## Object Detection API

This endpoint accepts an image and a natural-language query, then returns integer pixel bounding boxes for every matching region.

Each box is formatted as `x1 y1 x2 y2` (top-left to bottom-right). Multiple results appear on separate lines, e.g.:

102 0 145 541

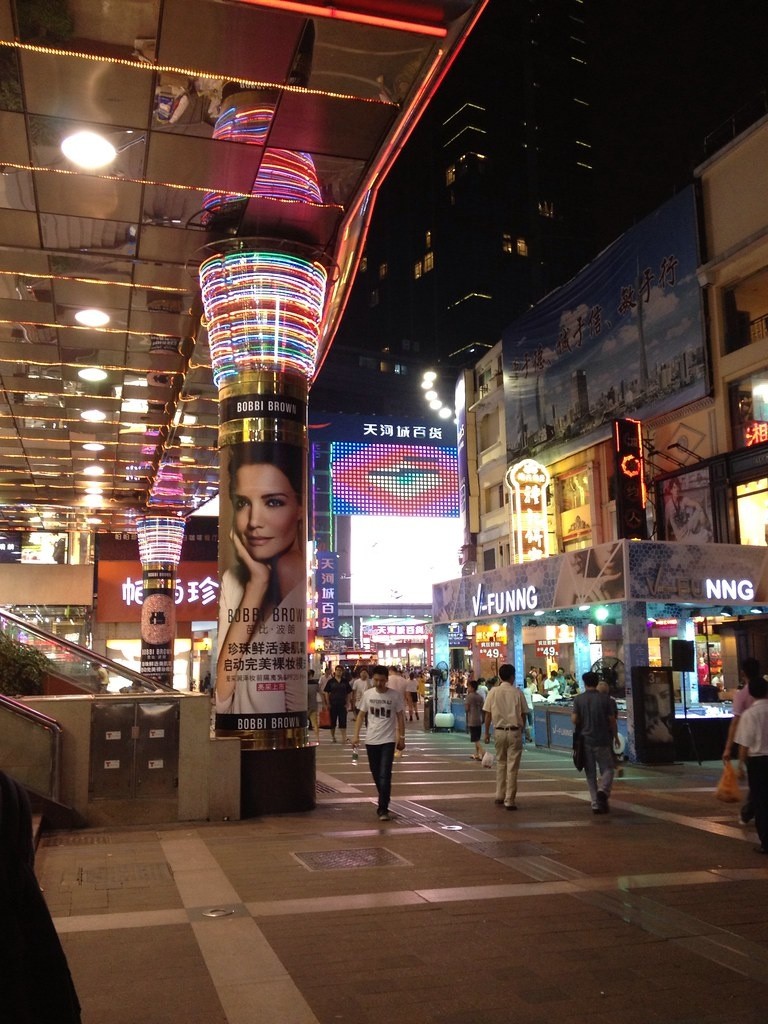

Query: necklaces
672 498 683 521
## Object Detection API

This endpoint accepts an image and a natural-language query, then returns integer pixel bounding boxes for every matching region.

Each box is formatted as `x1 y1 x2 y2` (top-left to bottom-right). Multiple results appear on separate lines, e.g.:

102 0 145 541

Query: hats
360 667 368 673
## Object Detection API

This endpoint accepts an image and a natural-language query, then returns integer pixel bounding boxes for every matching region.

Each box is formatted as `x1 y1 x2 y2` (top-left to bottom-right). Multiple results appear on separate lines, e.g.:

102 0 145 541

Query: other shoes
596 792 609 814
593 809 600 815
753 846 768 854
342 738 351 743
506 806 518 811
410 715 412 721
331 738 336 744
379 814 390 821
417 717 419 720
387 812 398 819
738 806 750 824
495 799 504 804
469 753 482 761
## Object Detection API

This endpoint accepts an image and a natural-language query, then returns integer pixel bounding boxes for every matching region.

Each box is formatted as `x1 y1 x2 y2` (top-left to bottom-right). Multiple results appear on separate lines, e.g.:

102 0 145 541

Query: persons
88 662 212 694
554 476 715 606
213 440 306 714
307 657 768 854
433 581 457 623
0 774 80 1024
645 673 673 755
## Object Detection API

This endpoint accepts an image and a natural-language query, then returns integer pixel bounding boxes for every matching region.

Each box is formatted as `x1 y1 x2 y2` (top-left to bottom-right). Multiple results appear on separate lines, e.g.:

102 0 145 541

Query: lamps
528 619 537 628
751 606 763 614
467 621 477 626
59 130 146 170
689 610 702 617
559 621 567 628
721 605 734 617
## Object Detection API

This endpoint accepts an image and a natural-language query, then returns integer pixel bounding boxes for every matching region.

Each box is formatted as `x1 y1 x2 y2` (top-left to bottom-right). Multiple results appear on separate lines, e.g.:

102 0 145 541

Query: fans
589 656 626 700
428 661 449 733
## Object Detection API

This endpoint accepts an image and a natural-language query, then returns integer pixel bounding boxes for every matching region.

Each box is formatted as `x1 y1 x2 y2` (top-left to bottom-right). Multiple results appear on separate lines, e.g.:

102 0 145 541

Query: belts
495 727 518 730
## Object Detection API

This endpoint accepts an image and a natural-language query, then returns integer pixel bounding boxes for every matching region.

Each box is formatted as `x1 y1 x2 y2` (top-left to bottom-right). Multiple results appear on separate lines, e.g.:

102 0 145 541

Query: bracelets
399 735 406 739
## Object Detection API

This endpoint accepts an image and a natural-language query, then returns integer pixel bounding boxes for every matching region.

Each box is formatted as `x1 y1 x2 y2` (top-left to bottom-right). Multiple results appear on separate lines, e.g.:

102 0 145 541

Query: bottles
352 744 358 766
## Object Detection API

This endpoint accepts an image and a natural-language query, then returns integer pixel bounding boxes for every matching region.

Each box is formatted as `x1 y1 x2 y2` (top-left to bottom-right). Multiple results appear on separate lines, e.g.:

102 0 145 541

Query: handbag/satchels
572 731 583 772
482 752 495 769
715 762 742 802
